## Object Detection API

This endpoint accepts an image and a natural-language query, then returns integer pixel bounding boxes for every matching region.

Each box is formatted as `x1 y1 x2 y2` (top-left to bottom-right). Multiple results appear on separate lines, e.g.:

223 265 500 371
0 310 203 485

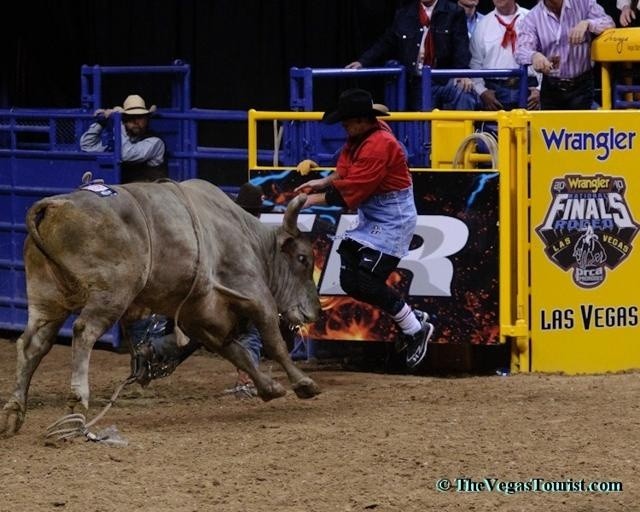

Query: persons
343 1 639 112
79 94 169 353
294 88 435 369
136 182 276 401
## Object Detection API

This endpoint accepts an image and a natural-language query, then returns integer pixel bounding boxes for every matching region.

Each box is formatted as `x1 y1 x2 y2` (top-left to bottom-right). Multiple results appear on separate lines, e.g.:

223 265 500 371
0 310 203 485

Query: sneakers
127 341 158 386
393 308 429 353
405 319 436 368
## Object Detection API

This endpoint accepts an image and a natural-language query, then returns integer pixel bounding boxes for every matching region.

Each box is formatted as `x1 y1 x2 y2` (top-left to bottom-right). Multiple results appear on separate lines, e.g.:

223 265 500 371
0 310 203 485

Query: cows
0 177 326 439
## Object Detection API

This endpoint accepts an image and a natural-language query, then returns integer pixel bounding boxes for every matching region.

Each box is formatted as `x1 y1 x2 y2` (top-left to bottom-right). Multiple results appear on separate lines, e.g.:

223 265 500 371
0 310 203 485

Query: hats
114 94 158 118
317 90 391 128
